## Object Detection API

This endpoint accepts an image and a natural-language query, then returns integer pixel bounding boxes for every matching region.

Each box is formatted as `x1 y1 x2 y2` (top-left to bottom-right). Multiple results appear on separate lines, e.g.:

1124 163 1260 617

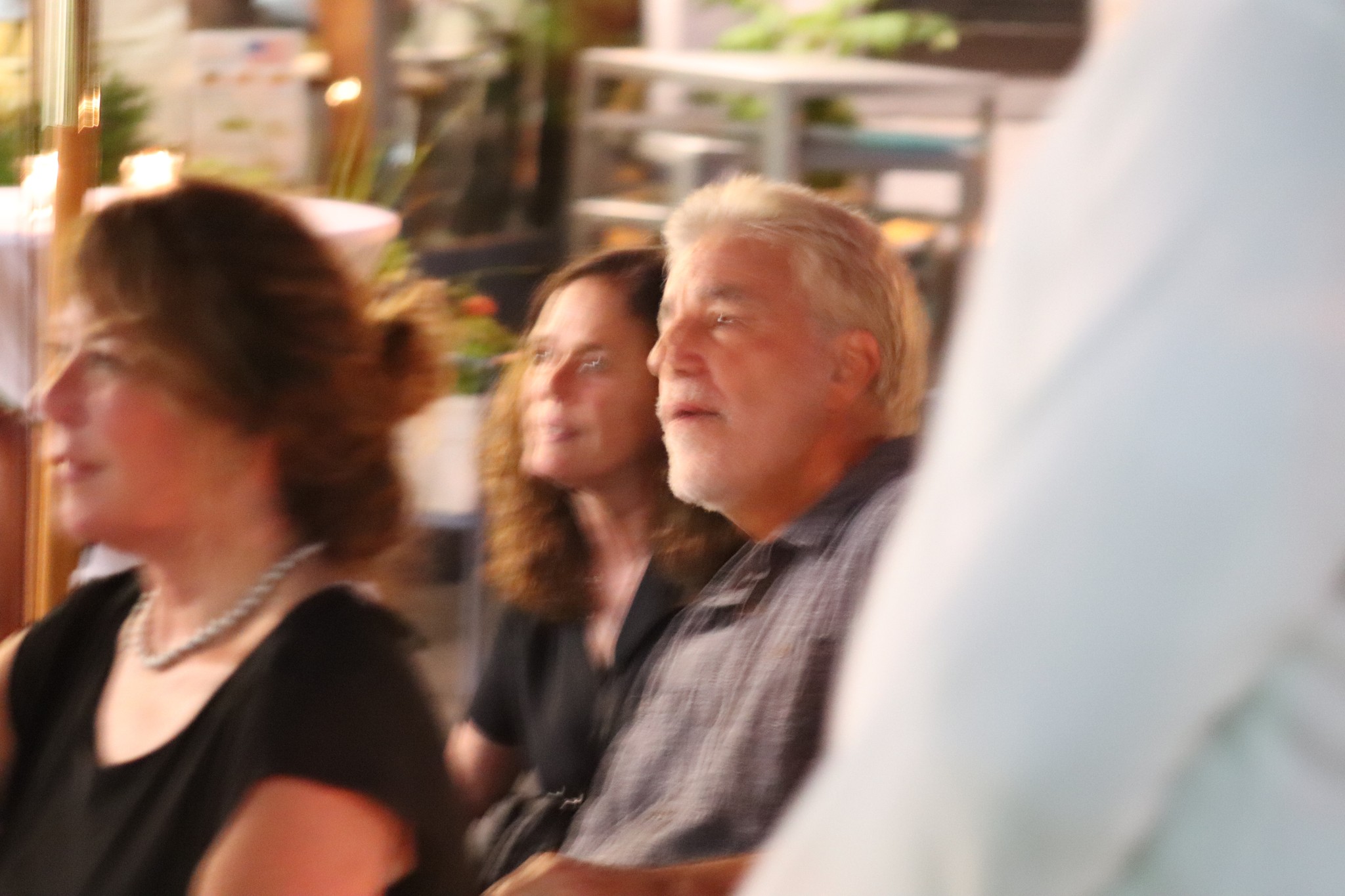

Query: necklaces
134 541 325 668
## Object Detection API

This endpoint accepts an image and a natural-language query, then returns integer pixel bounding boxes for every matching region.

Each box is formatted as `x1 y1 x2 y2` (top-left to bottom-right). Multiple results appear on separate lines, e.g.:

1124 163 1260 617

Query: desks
0 185 401 638
577 45 994 193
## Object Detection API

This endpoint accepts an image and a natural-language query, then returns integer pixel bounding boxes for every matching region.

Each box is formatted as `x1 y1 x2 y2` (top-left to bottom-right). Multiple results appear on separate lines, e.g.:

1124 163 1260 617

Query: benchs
569 198 955 257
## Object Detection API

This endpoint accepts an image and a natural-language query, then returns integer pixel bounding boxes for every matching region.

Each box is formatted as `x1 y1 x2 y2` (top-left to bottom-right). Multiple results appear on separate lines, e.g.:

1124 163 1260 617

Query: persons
726 0 1345 896
0 160 482 896
383 163 927 896
